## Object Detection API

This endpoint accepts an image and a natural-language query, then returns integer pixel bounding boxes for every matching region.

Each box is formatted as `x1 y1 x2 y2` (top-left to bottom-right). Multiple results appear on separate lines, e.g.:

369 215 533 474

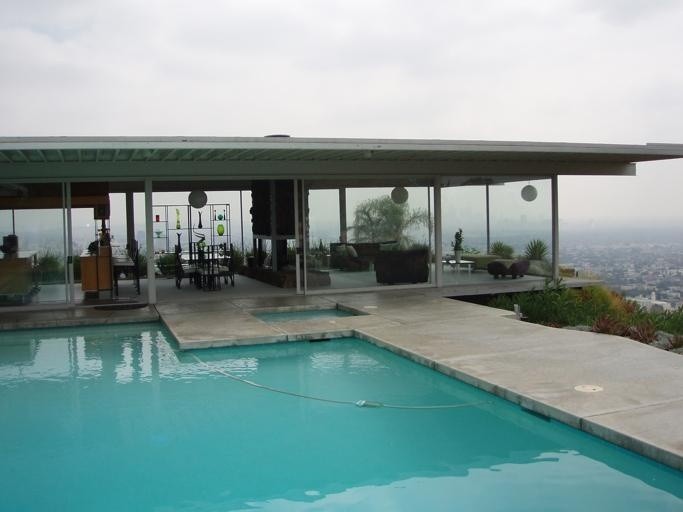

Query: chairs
112 238 142 297
173 240 235 293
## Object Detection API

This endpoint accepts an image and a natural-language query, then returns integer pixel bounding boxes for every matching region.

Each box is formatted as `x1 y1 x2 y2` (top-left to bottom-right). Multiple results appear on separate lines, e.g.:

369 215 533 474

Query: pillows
334 243 359 257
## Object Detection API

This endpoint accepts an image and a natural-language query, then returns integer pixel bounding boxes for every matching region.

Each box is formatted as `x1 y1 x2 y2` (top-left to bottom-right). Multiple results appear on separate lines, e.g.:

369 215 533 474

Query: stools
487 261 528 280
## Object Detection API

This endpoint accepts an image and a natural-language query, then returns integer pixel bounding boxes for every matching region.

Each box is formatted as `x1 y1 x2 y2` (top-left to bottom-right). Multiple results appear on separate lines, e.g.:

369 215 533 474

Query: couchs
374 247 430 285
329 242 400 272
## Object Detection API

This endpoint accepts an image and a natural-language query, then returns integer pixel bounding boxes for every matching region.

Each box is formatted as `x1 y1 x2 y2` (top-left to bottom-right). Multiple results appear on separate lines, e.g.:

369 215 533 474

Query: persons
175 245 185 288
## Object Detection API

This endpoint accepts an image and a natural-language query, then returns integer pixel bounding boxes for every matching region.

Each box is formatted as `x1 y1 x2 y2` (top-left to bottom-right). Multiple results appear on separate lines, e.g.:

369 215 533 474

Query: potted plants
448 226 467 262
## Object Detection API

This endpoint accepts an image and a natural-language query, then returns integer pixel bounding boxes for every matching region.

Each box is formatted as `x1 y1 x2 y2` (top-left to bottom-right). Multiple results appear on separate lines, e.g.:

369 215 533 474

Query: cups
197 243 203 251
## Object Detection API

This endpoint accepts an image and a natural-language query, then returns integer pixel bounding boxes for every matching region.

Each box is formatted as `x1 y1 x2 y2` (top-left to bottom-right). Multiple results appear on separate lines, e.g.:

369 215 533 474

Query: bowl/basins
155 231 162 237
112 254 129 263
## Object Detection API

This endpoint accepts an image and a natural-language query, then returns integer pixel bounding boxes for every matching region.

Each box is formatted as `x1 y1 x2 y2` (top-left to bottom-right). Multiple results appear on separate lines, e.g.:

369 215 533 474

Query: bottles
216 224 224 235
155 215 158 221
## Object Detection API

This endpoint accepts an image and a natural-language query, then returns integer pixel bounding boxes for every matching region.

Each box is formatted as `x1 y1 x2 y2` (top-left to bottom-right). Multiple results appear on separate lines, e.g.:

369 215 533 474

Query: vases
217 225 225 236
175 209 180 229
198 210 203 228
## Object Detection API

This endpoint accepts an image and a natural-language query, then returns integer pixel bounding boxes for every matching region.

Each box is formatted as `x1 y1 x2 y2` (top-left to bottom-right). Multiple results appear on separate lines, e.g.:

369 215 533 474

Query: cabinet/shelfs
150 203 233 253
0 249 44 308
